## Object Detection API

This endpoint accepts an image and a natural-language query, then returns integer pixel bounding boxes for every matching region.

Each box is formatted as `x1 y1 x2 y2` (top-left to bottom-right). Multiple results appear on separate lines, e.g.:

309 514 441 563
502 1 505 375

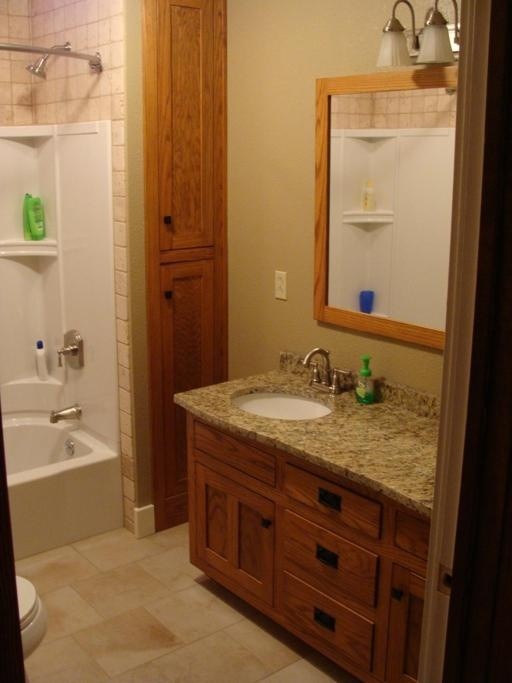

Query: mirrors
313 66 463 351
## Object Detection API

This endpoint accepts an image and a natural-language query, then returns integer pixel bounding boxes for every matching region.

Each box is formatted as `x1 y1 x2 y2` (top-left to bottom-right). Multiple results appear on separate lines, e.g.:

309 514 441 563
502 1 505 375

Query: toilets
16 573 48 662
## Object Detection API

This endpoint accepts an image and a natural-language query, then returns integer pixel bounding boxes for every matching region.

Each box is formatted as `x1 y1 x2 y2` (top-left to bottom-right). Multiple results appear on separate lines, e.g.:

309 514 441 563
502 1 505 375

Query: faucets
49 403 81 424
302 347 332 389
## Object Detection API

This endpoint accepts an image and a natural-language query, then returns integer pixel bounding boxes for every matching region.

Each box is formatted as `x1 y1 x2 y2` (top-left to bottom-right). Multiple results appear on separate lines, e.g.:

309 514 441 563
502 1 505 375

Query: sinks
229 384 333 419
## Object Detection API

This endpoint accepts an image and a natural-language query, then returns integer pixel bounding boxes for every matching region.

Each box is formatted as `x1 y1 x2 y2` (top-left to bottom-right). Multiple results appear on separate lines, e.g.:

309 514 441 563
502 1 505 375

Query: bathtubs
2 413 123 563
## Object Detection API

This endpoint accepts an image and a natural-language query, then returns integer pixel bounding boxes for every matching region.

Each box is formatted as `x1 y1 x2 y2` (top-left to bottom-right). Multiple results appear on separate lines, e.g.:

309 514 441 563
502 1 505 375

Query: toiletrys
362 177 377 212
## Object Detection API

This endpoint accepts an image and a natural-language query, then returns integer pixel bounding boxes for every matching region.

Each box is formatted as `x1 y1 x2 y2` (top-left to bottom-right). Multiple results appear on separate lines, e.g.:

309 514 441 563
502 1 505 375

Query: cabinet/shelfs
186 409 431 682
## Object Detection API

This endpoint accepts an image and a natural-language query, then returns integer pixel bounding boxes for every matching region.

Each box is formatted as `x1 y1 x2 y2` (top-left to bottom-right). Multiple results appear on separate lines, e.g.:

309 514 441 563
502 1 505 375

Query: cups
359 290 373 313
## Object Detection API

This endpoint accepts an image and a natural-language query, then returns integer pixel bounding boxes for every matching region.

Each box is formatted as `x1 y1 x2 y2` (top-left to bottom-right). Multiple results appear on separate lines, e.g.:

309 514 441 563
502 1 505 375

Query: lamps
376 0 461 68
